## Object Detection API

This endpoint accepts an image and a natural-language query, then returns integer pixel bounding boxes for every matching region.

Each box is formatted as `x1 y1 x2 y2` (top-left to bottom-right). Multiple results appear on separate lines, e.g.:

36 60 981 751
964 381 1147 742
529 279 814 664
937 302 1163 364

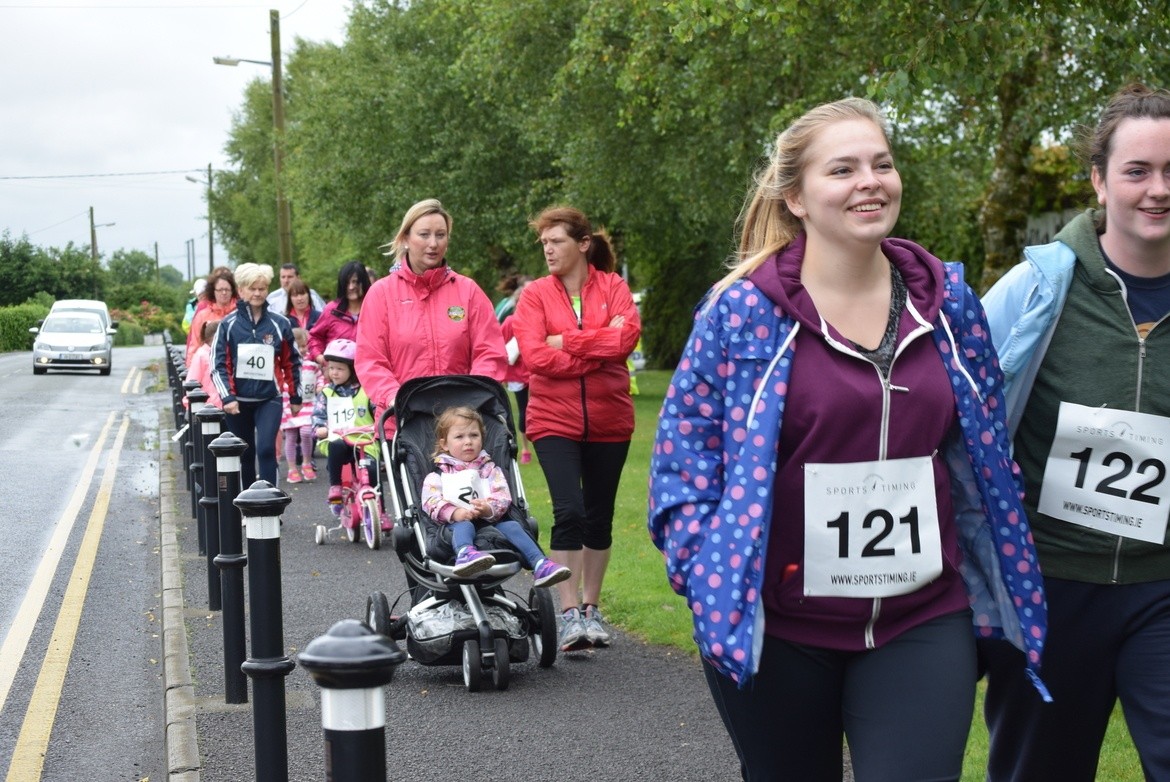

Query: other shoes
379 514 393 530
328 484 342 499
310 458 318 471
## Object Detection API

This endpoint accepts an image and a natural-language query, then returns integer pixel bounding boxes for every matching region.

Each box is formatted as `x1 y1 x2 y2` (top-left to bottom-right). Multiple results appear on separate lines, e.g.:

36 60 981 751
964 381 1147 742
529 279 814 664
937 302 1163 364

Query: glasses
215 287 232 293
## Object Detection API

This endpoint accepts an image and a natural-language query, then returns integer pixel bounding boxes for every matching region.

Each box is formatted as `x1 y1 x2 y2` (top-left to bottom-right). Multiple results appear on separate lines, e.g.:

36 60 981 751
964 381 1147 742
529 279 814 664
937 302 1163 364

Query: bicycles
314 428 390 551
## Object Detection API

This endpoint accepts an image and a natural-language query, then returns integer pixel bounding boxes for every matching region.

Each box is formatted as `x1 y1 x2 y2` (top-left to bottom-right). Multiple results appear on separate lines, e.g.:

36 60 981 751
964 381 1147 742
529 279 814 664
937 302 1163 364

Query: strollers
377 374 558 693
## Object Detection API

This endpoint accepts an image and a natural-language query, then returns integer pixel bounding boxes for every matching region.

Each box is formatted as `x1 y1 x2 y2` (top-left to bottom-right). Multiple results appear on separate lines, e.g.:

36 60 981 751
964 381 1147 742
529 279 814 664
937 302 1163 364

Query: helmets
323 339 357 366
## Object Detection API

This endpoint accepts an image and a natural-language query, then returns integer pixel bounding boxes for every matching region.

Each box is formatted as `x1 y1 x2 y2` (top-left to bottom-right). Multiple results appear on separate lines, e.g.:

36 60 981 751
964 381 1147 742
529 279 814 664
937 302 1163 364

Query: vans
38 298 119 352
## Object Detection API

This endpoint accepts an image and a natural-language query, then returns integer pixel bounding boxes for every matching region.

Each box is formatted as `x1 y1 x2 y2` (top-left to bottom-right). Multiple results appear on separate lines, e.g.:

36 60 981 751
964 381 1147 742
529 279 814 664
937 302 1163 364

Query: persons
208 263 305 503
182 258 379 485
308 337 397 533
496 205 642 652
646 94 1049 781
423 408 575 592
352 195 505 623
962 73 1170 782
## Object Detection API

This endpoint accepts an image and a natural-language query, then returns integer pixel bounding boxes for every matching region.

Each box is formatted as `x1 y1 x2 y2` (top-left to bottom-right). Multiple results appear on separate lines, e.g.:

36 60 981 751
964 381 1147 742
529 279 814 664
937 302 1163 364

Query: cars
28 312 117 376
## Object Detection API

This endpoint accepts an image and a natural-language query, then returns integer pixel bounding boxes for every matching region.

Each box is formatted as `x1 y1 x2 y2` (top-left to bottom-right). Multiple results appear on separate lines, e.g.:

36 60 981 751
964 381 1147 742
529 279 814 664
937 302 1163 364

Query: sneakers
286 468 303 484
302 463 317 480
581 603 612 647
557 605 592 651
532 559 571 587
453 546 497 577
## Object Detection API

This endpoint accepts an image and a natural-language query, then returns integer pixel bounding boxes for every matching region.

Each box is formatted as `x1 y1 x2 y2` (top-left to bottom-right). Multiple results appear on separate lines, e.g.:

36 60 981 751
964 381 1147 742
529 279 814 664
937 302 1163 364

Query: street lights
212 54 294 266
185 175 213 273
91 223 117 300
